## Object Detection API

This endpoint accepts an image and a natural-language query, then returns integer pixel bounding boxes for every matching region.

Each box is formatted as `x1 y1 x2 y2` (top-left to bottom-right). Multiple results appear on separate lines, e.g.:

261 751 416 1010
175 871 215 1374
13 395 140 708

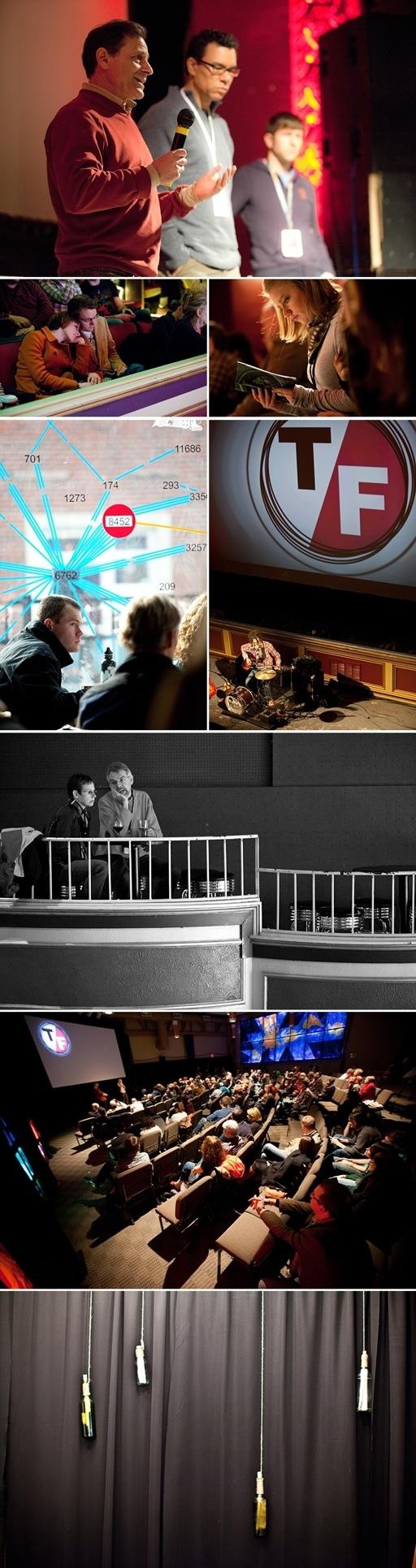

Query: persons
0 595 89 730
94 761 179 900
231 112 337 277
77 592 206 730
87 1067 416 1289
47 773 125 900
241 630 282 694
44 19 237 277
0 279 416 416
137 27 241 277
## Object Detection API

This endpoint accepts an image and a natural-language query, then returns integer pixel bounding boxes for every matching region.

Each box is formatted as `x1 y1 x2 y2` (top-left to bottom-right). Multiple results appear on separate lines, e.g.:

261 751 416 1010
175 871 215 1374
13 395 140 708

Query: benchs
1 322 153 405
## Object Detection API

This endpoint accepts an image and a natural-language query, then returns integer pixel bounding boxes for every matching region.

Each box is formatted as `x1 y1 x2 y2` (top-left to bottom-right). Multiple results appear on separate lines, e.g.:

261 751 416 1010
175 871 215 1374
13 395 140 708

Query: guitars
241 654 290 671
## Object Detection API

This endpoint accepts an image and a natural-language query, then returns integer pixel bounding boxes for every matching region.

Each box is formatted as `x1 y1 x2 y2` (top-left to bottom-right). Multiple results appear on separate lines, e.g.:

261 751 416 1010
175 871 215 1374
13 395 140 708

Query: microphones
160 108 195 188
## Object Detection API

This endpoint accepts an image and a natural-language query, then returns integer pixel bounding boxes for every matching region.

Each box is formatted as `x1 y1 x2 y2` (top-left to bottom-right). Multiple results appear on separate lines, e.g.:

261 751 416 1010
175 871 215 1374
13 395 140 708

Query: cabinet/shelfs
124 280 163 318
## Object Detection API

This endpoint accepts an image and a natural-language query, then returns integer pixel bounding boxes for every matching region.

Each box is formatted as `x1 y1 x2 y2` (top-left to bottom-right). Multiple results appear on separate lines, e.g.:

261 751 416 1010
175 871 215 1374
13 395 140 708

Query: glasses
79 790 98 794
199 59 240 78
79 313 99 322
309 1191 322 1205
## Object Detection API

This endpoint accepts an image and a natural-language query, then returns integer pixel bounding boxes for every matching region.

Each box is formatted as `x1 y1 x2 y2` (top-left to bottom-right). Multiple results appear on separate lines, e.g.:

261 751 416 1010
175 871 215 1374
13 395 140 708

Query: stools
133 873 151 898
60 868 235 897
289 899 398 932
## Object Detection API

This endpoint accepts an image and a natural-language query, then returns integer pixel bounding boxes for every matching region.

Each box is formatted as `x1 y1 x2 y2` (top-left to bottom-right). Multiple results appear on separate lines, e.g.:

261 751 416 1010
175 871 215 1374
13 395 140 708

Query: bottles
82 1374 96 1441
253 1471 267 1535
134 1345 150 1386
358 1351 373 1412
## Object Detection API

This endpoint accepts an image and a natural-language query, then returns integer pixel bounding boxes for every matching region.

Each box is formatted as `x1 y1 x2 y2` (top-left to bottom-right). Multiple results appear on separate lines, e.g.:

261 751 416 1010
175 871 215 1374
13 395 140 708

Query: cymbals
255 669 276 680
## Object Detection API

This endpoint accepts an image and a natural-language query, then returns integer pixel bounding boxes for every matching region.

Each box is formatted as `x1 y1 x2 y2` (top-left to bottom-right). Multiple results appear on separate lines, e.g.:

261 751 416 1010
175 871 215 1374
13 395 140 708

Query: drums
224 685 254 715
